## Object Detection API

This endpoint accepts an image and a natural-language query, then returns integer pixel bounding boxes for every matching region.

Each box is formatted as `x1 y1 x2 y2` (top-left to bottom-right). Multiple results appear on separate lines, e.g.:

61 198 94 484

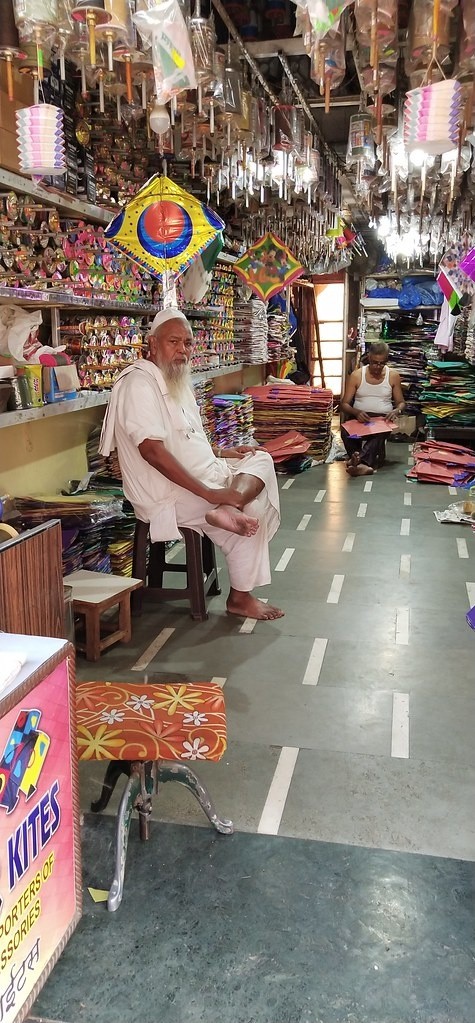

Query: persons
97 308 285 620
340 343 406 476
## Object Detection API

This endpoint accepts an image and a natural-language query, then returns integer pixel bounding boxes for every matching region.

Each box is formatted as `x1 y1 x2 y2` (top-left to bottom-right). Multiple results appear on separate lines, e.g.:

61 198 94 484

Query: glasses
369 358 385 367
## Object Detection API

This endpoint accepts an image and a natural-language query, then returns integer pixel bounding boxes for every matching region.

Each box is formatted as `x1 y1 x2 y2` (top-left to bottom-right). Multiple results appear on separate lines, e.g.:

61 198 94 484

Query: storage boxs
37 360 80 404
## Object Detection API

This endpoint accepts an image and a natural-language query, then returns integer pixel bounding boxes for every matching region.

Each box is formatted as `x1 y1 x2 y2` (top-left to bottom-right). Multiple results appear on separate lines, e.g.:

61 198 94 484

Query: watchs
397 408 403 413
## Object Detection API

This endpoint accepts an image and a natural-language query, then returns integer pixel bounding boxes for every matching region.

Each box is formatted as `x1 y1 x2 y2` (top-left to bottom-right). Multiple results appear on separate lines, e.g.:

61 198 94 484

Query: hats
147 309 195 336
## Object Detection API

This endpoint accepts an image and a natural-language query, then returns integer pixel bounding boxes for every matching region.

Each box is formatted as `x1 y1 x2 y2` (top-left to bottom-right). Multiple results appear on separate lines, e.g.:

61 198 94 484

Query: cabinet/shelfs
1 0 245 430
356 270 450 372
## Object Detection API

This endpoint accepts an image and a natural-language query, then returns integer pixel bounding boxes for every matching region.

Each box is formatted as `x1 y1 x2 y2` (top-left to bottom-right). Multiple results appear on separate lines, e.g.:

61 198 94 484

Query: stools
64 568 145 665
67 680 233 913
130 518 221 625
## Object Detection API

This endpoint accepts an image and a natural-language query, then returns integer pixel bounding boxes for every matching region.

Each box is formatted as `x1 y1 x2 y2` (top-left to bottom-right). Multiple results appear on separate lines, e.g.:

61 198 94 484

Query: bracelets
218 449 223 457
